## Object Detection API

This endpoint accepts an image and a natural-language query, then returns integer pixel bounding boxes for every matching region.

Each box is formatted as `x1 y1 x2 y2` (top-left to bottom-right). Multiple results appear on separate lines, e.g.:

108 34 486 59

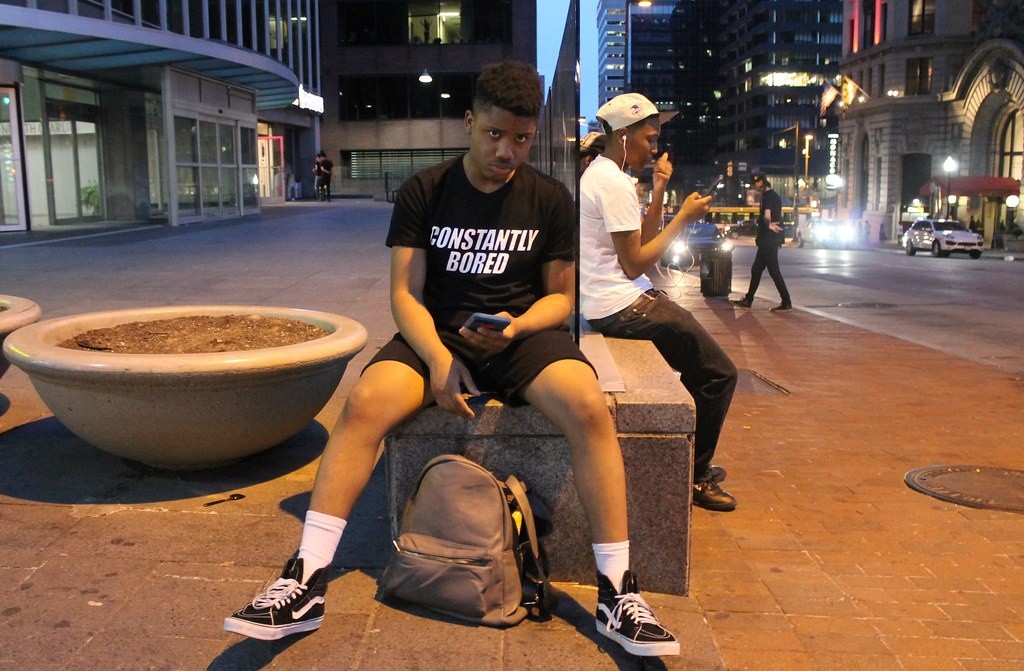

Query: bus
705 207 821 240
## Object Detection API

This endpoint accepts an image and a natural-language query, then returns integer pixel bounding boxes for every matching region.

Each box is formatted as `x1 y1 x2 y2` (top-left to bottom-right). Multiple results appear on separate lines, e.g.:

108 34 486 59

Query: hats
595 92 680 133
579 129 609 158
748 170 766 184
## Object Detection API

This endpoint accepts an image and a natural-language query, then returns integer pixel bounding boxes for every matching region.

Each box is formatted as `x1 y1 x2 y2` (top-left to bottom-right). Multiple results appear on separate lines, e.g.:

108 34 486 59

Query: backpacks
379 453 553 629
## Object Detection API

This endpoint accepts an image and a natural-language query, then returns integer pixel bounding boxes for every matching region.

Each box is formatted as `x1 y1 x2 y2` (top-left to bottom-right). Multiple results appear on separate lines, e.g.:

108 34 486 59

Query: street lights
805 134 815 180
623 0 653 93
356 104 372 121
944 155 959 219
420 66 444 164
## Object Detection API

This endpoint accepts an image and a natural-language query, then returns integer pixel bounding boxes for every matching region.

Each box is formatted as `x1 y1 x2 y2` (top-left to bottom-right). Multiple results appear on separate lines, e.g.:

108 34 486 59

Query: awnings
918 177 1020 217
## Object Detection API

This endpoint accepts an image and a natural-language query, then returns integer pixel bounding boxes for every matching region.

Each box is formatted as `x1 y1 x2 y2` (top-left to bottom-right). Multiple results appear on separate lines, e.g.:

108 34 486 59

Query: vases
0 293 367 469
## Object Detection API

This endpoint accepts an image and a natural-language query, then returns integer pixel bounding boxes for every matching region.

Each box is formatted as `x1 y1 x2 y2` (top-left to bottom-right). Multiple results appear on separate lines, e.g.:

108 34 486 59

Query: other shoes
709 465 726 481
693 477 739 509
768 304 792 312
729 296 751 310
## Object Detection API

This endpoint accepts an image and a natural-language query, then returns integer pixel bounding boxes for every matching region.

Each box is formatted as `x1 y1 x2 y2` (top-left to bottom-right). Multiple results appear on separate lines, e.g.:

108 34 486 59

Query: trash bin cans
699 244 733 297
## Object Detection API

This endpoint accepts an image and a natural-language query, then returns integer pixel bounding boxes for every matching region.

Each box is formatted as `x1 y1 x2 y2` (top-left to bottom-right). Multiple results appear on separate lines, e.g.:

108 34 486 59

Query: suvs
684 223 732 265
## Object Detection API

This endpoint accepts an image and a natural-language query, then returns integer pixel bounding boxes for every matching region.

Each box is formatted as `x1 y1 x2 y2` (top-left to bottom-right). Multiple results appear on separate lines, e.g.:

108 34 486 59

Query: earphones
620 136 627 146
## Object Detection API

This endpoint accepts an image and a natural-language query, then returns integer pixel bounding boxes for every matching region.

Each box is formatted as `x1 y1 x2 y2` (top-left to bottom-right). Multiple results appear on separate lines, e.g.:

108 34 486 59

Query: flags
840 75 858 118
819 81 839 116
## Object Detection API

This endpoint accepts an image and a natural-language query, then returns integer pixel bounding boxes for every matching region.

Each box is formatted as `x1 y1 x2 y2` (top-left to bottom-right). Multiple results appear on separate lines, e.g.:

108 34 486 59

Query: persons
896 222 903 246
863 220 871 244
221 63 681 657
968 214 976 232
580 91 738 512
311 150 334 202
727 172 793 312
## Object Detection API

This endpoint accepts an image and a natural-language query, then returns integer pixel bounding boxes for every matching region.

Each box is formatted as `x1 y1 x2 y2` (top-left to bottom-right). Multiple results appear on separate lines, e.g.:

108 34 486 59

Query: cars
726 219 759 239
903 218 984 259
797 218 854 249
664 213 675 227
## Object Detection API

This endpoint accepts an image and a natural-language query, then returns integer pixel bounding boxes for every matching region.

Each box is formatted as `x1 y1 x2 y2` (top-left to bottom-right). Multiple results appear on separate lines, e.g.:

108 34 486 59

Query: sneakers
594 568 680 656
222 549 332 641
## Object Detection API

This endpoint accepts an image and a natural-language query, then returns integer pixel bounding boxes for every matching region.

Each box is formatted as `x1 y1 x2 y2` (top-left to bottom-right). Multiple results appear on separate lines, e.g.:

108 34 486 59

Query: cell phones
701 175 724 197
464 313 511 334
